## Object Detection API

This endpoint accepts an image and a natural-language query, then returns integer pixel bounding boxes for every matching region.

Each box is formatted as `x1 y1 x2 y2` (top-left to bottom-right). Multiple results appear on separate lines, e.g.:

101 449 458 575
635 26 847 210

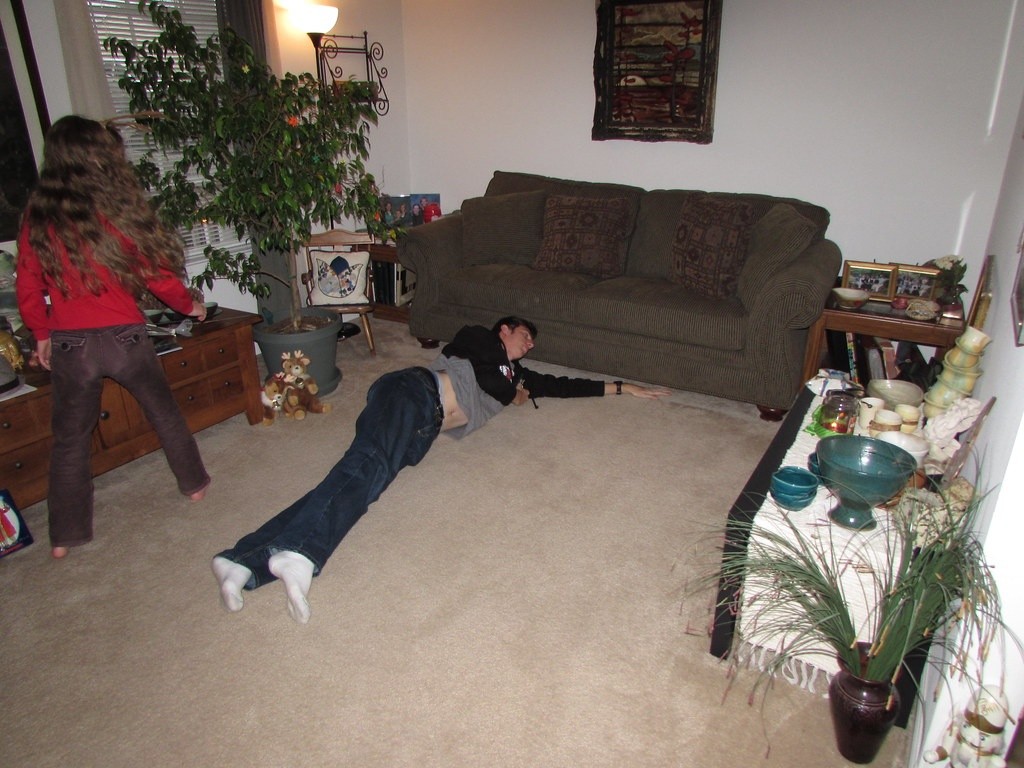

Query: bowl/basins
140 310 163 324
767 465 820 512
867 379 924 412
831 287 872 311
164 302 218 322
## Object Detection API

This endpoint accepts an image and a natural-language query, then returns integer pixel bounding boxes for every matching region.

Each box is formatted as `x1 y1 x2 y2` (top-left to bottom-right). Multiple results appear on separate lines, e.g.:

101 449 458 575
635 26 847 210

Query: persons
19 117 213 557
385 198 439 231
212 315 671 623
857 273 923 296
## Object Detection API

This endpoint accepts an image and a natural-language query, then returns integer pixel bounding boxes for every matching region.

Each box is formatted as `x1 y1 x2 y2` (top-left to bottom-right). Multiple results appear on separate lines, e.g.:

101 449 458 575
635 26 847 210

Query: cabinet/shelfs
0 307 264 508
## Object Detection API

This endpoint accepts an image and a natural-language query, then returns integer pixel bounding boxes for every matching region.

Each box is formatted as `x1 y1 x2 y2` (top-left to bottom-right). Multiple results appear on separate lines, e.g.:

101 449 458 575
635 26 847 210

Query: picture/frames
841 260 941 303
1009 242 1024 346
967 255 993 331
591 0 723 145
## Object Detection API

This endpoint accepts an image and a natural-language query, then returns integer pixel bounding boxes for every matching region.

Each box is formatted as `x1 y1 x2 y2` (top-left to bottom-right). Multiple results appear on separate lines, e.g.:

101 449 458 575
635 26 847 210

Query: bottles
817 388 861 437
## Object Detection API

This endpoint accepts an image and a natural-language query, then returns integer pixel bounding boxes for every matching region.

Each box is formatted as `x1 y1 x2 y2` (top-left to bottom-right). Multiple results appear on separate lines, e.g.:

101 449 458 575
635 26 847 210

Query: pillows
666 193 754 300
461 190 546 268
533 194 641 279
736 201 817 312
306 251 371 306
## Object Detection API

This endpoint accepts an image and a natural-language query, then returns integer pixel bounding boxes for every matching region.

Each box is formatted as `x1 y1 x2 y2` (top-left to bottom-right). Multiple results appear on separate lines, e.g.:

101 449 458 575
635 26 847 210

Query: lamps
290 6 361 340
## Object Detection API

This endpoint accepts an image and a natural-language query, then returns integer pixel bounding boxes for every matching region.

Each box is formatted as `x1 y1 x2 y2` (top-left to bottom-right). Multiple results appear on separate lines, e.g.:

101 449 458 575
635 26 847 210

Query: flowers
933 255 968 299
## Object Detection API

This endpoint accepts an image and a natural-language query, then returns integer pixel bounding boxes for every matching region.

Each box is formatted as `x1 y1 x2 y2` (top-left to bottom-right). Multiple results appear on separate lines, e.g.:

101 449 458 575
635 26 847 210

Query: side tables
356 229 411 325
800 277 966 397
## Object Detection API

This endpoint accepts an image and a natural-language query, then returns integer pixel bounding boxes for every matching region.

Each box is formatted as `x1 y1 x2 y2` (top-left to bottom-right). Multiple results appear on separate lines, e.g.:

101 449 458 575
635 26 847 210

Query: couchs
397 169 842 422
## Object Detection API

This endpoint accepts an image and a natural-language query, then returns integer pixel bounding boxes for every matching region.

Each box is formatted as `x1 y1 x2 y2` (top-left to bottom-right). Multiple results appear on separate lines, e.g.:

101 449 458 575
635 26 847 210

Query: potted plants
103 0 382 400
665 436 1024 763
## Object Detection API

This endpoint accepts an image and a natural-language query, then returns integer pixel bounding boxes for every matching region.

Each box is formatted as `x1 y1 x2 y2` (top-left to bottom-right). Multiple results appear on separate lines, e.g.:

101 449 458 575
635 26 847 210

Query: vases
941 285 967 319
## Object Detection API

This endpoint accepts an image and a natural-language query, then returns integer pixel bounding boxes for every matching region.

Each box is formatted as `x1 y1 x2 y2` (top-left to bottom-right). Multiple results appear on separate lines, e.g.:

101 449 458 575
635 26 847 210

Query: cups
922 325 993 423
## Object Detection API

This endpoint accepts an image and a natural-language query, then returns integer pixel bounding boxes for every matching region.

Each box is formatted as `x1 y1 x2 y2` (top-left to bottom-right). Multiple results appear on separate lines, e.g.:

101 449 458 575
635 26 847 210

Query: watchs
613 380 624 395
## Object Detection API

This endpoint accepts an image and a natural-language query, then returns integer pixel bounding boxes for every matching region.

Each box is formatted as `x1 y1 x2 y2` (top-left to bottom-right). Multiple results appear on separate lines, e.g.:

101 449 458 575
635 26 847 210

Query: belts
415 364 444 434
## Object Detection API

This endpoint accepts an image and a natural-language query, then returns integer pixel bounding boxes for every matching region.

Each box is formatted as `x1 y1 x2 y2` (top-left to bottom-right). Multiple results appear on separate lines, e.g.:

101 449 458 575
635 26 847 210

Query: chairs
302 229 378 357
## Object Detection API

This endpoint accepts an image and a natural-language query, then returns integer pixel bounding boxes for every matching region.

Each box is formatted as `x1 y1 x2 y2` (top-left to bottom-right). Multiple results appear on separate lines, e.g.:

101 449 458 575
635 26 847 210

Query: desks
709 386 956 728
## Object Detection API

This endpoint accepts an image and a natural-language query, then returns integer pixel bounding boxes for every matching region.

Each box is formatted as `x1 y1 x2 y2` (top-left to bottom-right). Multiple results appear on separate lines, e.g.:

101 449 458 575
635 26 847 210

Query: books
845 332 900 387
